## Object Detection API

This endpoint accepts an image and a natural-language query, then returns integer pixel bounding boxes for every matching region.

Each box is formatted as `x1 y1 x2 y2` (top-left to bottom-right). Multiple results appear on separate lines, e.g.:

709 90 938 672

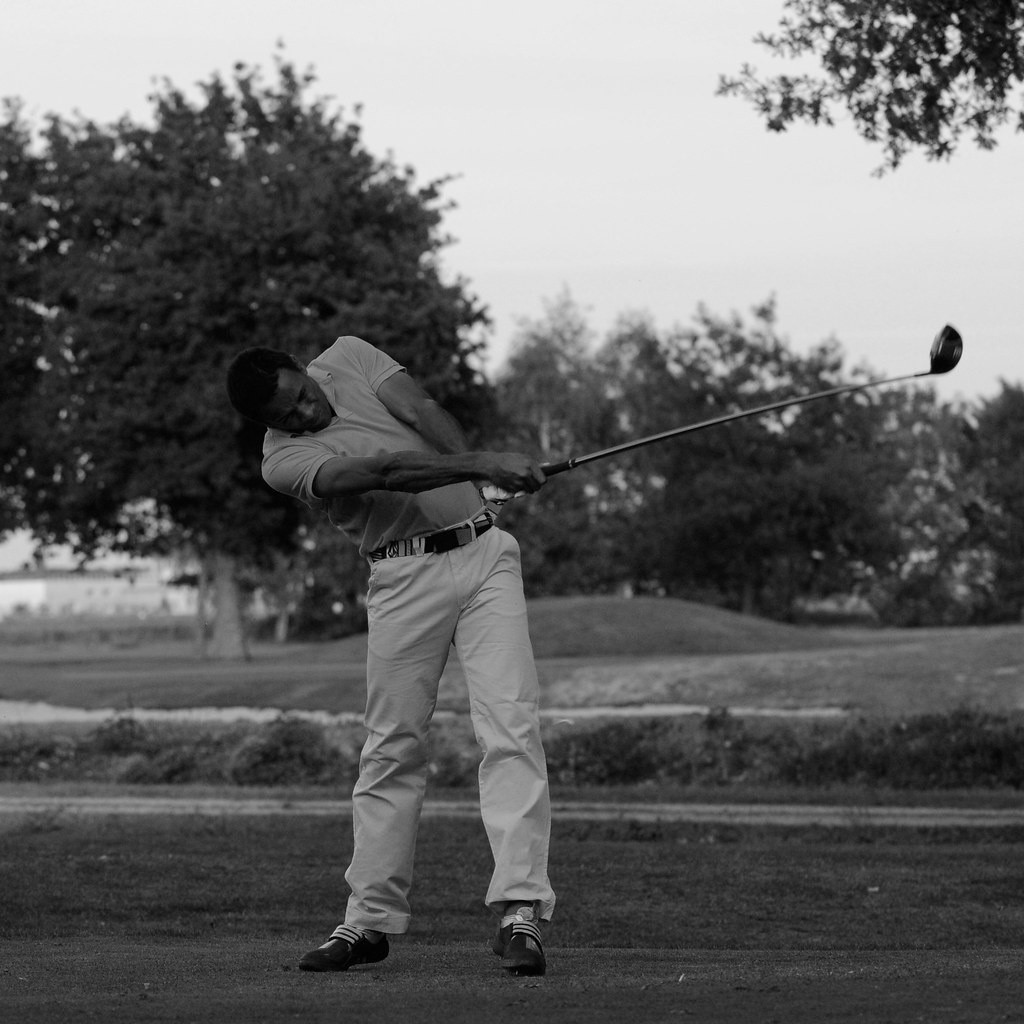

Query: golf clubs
478 323 964 501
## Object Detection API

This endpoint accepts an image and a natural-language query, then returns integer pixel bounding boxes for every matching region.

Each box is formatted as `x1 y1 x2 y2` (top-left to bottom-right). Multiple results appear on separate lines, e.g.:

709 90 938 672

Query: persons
229 334 557 975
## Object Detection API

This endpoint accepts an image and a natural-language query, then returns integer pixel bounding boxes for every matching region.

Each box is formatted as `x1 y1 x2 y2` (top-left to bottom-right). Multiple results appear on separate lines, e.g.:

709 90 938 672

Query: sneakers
298 924 389 972
492 907 547 977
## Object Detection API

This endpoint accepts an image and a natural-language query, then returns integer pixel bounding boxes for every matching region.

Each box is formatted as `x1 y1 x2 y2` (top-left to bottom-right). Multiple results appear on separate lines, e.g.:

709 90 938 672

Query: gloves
481 485 527 504
